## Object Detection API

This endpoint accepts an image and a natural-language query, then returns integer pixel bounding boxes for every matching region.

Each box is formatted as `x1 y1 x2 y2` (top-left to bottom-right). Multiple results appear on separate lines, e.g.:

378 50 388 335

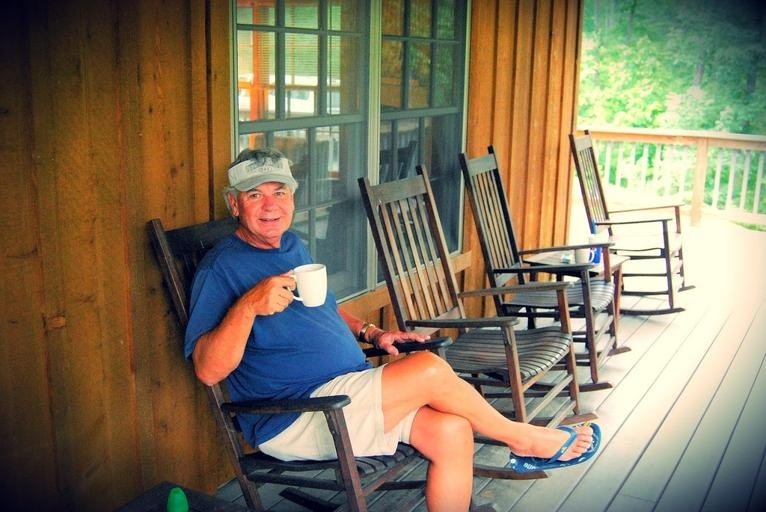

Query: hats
227 157 299 194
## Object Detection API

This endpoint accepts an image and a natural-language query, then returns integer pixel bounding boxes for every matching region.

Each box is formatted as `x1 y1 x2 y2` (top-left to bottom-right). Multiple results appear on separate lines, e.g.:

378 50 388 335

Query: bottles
589 219 602 264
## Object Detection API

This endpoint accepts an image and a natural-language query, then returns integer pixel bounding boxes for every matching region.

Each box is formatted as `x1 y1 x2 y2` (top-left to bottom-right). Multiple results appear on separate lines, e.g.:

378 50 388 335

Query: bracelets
358 319 377 345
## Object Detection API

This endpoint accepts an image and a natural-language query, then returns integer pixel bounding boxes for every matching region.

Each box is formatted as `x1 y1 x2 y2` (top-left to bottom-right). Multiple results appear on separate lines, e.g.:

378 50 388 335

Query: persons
183 146 602 512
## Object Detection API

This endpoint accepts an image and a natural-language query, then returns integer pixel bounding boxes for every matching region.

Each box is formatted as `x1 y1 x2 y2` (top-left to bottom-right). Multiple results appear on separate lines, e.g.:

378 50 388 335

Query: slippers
509 421 601 474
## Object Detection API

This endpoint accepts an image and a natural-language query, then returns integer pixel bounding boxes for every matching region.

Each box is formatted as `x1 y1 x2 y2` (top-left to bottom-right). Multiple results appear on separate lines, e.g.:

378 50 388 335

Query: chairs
357 163 597 448
147 216 548 511
459 144 631 392
569 130 695 316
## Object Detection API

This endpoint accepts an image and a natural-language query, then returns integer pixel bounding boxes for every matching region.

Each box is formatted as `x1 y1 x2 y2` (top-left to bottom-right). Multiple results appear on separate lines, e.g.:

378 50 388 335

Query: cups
286 263 328 308
573 248 595 263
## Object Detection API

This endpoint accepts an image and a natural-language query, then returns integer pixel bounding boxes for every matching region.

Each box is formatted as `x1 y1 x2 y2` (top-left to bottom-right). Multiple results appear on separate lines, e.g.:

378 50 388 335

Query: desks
113 481 262 512
